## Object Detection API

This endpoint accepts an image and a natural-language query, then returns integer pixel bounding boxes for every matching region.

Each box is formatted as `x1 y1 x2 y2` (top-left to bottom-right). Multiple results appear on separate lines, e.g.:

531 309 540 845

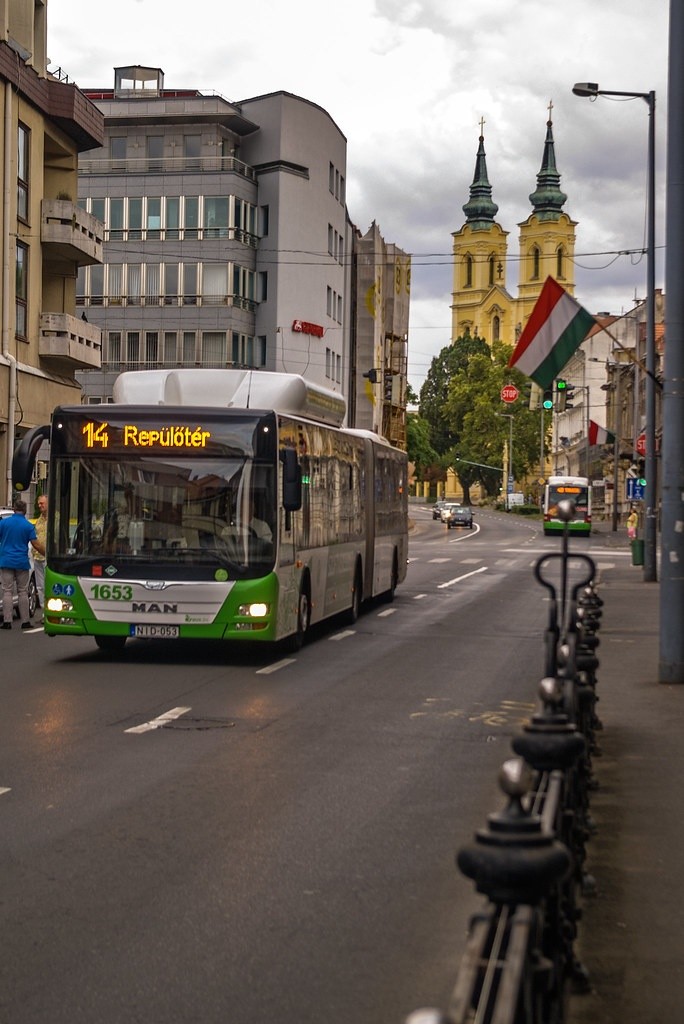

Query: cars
446 505 476 529
0 506 39 619
431 501 450 520
439 502 461 523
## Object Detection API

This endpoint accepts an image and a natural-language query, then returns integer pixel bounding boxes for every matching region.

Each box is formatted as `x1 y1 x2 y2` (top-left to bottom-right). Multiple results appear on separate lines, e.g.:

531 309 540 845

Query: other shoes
21 621 34 629
0 622 12 629
41 617 44 624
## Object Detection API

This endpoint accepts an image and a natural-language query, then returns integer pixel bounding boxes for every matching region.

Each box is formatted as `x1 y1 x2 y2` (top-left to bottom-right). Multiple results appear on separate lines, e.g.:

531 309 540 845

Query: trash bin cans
631 540 644 565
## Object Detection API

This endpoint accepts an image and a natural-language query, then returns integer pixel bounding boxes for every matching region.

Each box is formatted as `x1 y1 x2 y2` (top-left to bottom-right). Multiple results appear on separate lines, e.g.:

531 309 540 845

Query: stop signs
635 433 659 458
501 385 518 403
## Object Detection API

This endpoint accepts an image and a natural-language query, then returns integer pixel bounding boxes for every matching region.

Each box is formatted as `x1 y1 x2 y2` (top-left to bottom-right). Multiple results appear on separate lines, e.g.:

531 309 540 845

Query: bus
11 367 410 654
541 476 594 536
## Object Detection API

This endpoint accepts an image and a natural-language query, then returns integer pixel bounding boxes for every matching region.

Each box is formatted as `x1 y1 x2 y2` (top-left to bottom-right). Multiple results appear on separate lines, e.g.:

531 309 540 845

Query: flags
589 419 616 446
508 275 597 391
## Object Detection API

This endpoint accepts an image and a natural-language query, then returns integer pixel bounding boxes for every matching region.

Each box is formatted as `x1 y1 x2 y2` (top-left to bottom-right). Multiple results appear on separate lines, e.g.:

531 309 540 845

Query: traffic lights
362 368 377 383
456 451 460 462
556 378 566 392
637 458 647 487
542 390 553 410
522 383 539 411
559 384 575 412
384 375 401 407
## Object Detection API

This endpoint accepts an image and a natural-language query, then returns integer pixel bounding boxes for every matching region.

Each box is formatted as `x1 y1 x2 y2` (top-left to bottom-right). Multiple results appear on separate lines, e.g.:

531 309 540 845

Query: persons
221 496 272 542
0 500 46 630
31 495 60 622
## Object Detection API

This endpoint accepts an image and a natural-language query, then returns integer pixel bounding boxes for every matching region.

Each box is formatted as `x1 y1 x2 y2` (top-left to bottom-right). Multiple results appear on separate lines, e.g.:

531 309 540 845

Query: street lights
571 81 659 586
501 413 513 494
